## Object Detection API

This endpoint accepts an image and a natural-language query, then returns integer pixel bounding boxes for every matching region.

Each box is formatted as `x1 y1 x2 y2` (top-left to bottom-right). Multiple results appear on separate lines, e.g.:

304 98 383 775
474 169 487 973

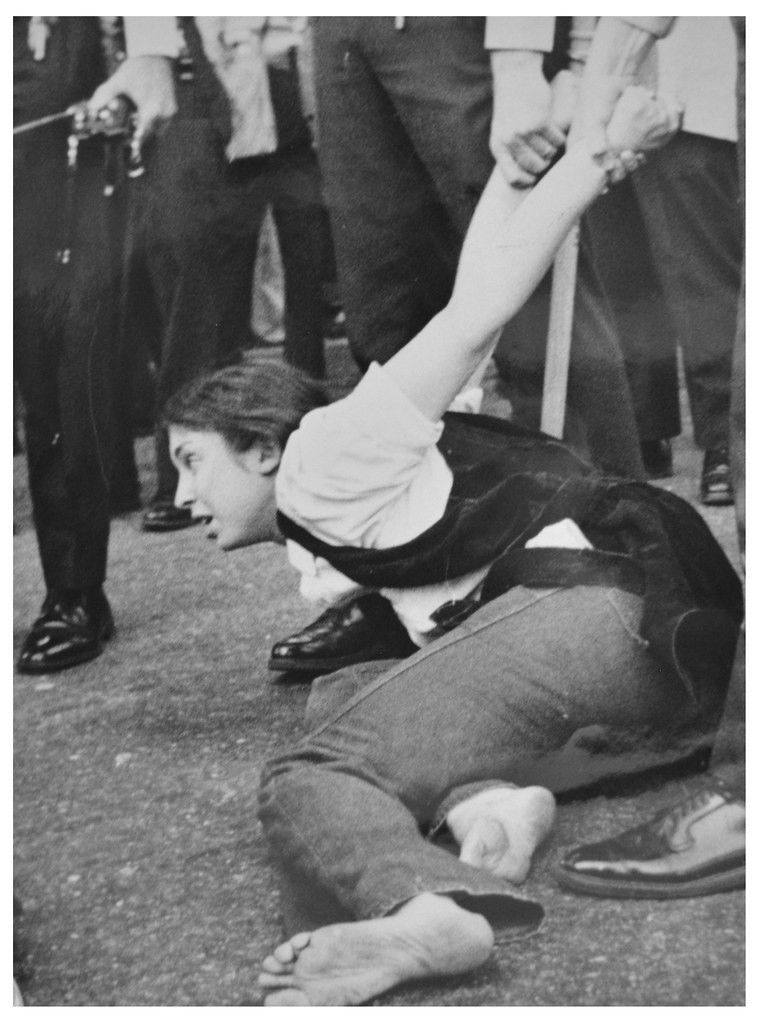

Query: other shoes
322 311 348 341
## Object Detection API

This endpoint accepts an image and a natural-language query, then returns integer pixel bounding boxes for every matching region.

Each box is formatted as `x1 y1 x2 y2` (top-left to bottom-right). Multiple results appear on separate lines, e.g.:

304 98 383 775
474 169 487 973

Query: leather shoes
550 772 746 899
141 493 195 529
702 442 734 504
16 584 113 670
638 435 672 478
269 593 424 672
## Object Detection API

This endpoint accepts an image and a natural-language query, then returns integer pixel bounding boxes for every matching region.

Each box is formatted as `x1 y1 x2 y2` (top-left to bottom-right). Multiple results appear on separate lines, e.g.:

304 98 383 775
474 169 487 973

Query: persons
102 16 734 674
481 18 746 895
162 69 746 1005
13 18 189 672
